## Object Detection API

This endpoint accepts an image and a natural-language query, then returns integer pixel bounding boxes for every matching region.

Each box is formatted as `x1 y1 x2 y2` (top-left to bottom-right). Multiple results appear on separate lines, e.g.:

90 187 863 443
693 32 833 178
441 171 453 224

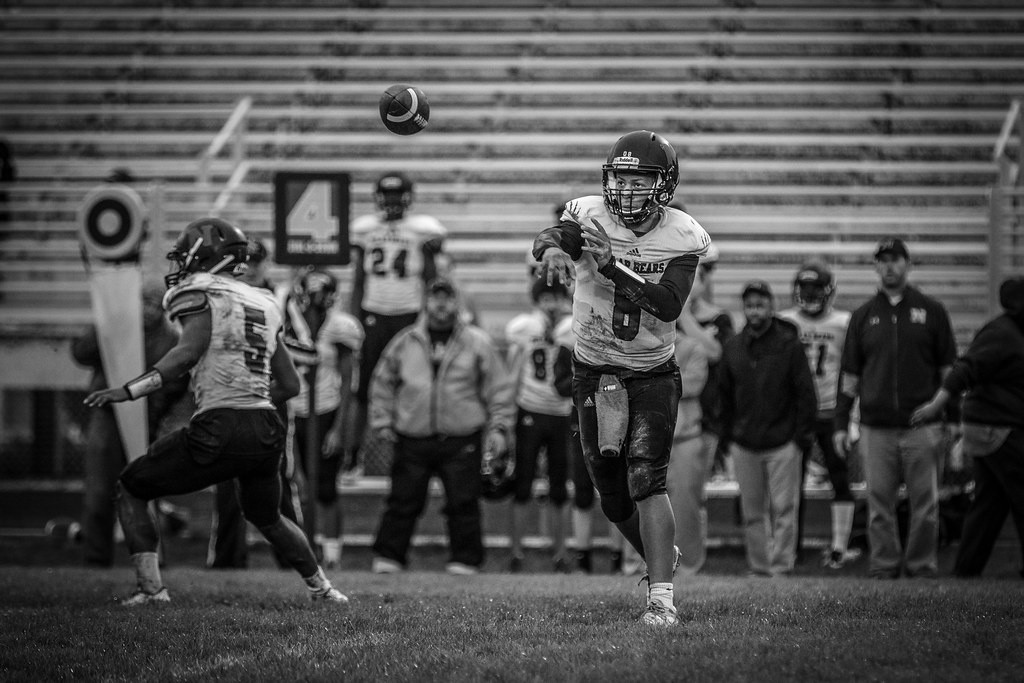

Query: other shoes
509 558 522 572
830 548 862 569
554 559 570 573
323 560 341 571
372 559 400 572
444 561 479 576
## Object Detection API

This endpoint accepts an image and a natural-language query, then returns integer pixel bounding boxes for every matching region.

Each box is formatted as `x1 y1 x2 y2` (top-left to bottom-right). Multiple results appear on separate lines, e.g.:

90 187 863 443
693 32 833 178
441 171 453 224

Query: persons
911 277 1023 578
683 252 734 475
345 171 436 471
290 270 366 568
83 218 348 608
775 267 864 576
507 275 576 571
529 129 710 624
366 283 517 574
618 335 710 574
833 239 959 578
204 238 330 571
716 283 817 576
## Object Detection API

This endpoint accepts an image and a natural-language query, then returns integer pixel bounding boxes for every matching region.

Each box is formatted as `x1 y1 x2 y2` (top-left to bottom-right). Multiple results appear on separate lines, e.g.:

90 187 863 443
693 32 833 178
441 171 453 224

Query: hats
740 279 776 302
873 238 910 260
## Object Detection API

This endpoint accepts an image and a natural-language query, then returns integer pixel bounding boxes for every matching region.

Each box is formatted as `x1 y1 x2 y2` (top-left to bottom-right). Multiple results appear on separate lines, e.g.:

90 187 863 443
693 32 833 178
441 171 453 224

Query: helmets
791 265 835 317
294 270 338 309
373 173 412 223
529 268 568 303
600 130 678 226
421 277 462 327
246 238 267 272
163 219 251 289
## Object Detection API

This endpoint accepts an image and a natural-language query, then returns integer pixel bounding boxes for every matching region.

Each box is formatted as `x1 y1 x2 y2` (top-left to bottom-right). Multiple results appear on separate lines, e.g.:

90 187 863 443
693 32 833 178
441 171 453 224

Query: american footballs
379 85 430 135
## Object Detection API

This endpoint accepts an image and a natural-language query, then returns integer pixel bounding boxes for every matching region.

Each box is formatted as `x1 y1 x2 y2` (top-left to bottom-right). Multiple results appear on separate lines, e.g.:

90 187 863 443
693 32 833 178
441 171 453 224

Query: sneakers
119 586 170 605
307 580 349 605
636 599 680 626
637 545 683 605
